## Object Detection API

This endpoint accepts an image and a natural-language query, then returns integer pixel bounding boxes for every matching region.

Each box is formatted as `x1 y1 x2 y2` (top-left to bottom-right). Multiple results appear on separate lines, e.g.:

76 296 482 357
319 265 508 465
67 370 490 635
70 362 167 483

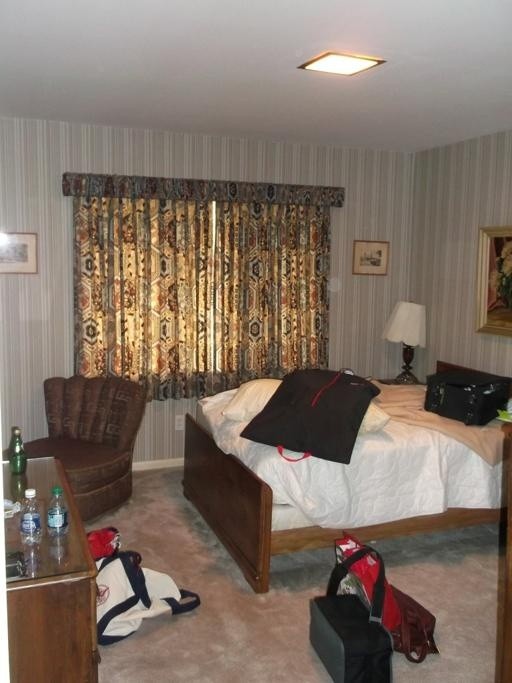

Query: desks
2 456 101 683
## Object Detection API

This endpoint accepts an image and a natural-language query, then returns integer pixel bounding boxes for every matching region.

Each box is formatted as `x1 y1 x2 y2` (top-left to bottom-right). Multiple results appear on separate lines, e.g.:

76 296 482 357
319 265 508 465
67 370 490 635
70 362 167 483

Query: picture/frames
0 232 38 273
474 225 512 337
352 239 390 275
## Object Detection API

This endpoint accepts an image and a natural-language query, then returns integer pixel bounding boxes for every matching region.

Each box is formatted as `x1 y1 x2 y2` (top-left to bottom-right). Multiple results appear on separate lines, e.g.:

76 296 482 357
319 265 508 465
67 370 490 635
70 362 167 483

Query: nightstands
378 377 425 384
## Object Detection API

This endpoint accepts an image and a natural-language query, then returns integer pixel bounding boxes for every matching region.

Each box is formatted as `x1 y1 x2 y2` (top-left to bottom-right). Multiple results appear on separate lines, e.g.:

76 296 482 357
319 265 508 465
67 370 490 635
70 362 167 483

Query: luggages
425 367 510 426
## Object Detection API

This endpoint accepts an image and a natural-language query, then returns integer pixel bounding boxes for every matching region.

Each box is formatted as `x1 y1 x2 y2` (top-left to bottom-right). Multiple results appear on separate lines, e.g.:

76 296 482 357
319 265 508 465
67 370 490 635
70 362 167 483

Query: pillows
220 378 391 433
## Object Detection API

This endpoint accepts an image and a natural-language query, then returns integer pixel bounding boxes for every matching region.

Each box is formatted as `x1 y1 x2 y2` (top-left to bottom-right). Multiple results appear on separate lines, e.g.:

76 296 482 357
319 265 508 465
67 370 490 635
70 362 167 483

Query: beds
179 360 511 593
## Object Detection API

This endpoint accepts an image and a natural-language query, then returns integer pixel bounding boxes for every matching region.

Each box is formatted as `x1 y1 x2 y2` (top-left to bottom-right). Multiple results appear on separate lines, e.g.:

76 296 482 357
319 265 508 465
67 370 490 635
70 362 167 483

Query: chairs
0 373 147 520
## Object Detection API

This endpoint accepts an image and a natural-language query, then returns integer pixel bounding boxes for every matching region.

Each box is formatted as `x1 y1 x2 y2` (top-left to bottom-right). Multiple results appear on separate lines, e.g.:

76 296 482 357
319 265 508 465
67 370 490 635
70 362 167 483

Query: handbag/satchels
311 548 394 683
390 583 439 662
95 553 200 645
87 526 121 559
239 367 381 466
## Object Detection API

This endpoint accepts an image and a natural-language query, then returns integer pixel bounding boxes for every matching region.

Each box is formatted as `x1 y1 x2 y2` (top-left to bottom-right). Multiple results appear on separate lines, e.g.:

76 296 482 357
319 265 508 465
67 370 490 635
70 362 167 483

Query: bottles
10 472 28 506
8 425 29 475
20 543 44 578
45 483 70 539
18 488 42 547
46 532 72 570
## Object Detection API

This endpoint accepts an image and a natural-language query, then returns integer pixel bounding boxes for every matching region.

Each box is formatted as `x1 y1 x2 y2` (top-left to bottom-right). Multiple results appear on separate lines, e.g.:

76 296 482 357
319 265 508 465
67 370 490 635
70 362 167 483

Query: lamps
379 300 427 384
